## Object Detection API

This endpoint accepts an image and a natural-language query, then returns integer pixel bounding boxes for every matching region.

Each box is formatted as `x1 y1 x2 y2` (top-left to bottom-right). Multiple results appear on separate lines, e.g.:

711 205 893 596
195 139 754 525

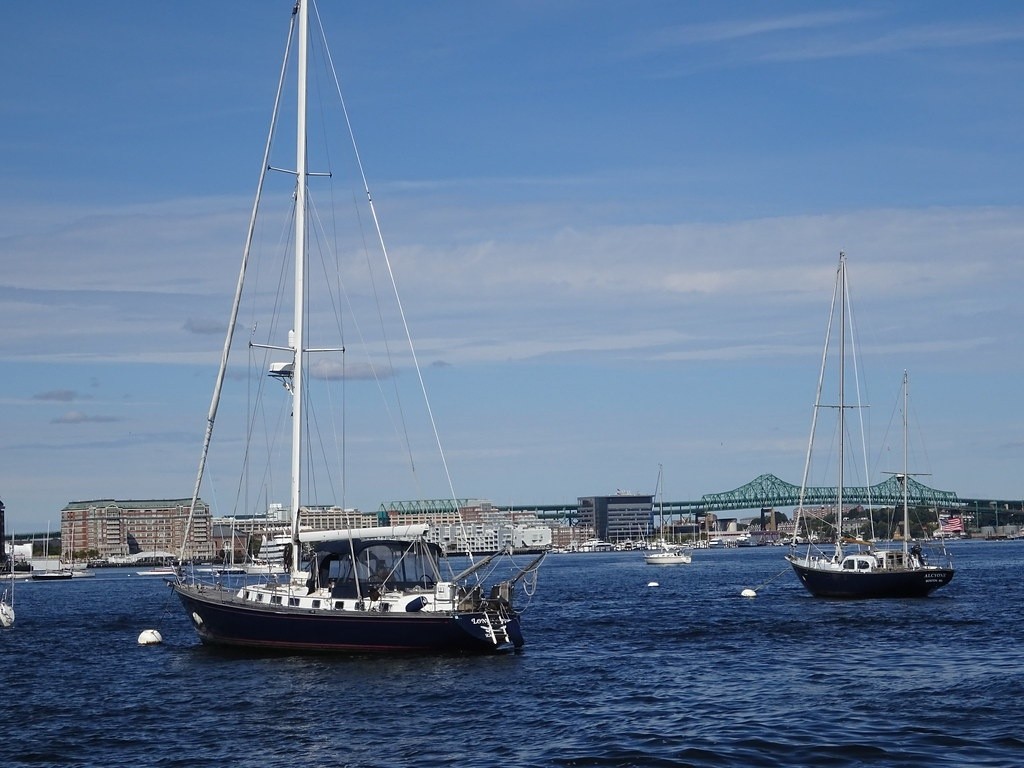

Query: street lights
989 502 998 526
974 500 979 529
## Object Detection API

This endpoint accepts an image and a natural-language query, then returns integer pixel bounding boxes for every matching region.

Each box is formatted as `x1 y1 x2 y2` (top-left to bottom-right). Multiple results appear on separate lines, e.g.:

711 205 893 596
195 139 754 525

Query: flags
940 518 963 531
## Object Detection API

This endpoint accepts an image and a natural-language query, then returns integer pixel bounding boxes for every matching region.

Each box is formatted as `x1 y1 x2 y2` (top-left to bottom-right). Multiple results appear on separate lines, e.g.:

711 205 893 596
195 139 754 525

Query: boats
736 532 758 547
136 519 187 576
246 534 292 576
547 523 724 554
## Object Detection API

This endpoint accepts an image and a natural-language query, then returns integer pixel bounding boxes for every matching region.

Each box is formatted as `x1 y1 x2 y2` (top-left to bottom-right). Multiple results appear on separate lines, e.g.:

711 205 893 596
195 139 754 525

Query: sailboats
161 0 554 653
785 249 957 600
0 519 95 581
642 462 694 564
0 528 17 629
216 517 245 574
